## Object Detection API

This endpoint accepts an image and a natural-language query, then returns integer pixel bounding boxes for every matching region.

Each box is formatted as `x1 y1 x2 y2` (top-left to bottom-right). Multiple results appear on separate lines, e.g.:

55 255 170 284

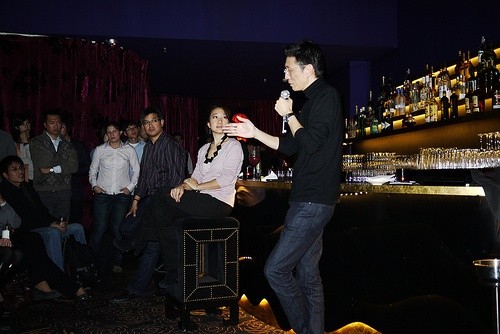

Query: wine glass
248 147 260 181
341 131 500 184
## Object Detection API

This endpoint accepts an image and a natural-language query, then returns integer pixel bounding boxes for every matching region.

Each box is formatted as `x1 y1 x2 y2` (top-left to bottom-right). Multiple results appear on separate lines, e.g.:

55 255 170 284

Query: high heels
113 237 147 255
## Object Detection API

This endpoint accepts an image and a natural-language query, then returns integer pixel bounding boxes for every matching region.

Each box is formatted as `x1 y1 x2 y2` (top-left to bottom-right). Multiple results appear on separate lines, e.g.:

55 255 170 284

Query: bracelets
133 198 138 201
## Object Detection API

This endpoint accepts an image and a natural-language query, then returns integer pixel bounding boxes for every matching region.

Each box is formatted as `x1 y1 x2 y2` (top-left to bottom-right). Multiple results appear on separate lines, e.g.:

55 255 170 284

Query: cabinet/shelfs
342 47 500 140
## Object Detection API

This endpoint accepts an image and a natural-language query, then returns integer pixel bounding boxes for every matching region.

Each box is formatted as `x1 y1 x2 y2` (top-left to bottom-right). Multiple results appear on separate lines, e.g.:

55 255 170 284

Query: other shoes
110 265 123 280
33 288 62 299
108 289 142 303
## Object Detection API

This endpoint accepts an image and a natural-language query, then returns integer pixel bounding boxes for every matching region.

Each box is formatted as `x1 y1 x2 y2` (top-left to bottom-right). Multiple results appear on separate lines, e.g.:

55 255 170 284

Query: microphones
280 90 290 134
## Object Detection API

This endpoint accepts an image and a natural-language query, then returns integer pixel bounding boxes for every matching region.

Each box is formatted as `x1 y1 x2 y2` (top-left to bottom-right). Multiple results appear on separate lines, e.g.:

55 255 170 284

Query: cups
56 216 68 231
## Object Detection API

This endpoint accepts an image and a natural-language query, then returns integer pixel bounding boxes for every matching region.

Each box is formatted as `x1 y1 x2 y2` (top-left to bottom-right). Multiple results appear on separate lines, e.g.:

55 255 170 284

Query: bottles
345 37 500 139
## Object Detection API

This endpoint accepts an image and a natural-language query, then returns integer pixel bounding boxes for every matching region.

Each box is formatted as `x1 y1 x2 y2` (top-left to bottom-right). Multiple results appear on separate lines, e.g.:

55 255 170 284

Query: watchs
285 113 295 122
50 167 53 172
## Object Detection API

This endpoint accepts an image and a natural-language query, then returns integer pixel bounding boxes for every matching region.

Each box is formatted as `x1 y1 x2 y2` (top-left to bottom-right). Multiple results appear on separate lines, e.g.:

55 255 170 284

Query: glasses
284 62 300 74
144 119 160 124
126 127 137 131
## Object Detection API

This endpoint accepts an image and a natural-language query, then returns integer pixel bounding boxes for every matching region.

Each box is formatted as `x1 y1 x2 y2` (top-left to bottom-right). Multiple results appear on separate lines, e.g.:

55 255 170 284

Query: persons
221 40 345 334
0 104 244 303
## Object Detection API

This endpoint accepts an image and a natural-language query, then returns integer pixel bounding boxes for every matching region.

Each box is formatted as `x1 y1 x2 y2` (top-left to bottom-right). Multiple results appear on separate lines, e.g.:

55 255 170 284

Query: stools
165 215 240 330
